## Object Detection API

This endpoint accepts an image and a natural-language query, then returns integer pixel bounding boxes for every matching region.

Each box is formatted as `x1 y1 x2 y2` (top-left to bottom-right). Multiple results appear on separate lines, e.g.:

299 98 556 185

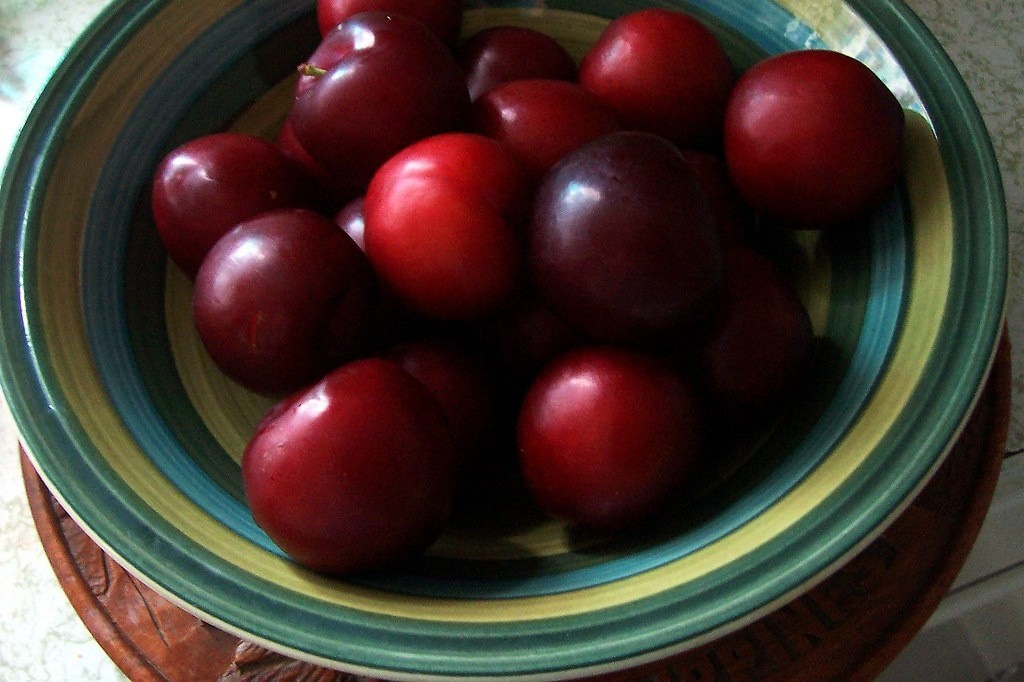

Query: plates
0 0 1012 682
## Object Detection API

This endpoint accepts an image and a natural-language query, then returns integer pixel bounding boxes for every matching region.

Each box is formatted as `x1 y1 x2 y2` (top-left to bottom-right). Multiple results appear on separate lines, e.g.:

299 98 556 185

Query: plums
149 0 910 569
